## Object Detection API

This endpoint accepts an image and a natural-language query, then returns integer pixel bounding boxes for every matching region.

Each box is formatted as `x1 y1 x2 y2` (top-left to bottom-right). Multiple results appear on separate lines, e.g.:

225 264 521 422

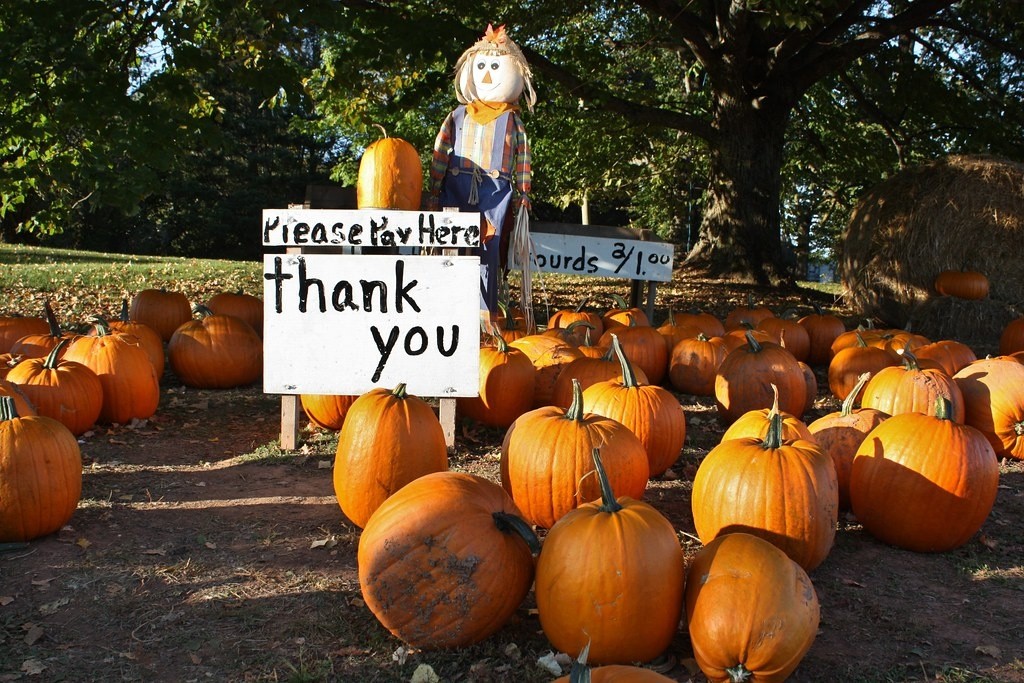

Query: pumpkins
356 123 422 210
296 264 1024 683
0 286 265 542
